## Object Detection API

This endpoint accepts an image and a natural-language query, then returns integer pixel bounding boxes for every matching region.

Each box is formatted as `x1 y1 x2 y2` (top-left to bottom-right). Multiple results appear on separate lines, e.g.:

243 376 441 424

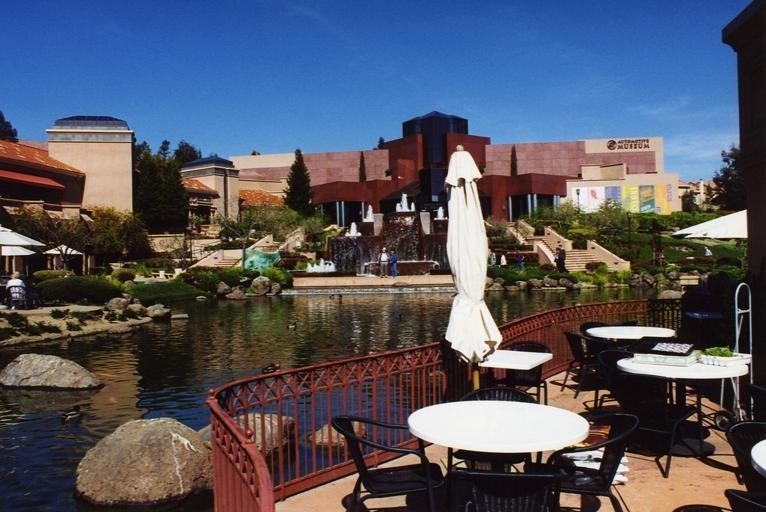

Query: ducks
286 320 297 330
61 404 81 422
261 360 282 374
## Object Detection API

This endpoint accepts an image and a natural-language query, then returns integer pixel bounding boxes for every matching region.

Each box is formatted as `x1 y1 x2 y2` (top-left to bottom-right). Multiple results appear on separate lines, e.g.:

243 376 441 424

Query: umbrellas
671 209 749 240
0 224 47 246
0 245 36 256
443 144 503 399
41 244 83 269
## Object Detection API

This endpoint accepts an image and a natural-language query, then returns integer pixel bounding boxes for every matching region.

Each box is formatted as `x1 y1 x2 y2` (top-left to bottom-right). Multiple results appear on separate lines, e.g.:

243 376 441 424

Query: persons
5 271 26 310
500 251 508 268
377 247 390 278
554 247 561 271
388 250 399 278
657 248 665 258
517 250 525 270
557 245 570 273
491 252 496 267
704 246 714 256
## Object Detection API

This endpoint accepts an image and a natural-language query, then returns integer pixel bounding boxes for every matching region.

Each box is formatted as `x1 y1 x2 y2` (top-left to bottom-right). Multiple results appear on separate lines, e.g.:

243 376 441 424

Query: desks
407 399 592 473
749 440 766 474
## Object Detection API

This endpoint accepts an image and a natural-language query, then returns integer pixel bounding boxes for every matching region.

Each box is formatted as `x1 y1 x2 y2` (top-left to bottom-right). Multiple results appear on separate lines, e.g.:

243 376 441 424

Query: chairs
598 368 704 478
472 321 766 438
447 463 561 512
723 487 766 511
546 411 641 512
6 286 34 310
329 411 445 512
671 503 735 512
725 421 766 494
453 384 539 469
